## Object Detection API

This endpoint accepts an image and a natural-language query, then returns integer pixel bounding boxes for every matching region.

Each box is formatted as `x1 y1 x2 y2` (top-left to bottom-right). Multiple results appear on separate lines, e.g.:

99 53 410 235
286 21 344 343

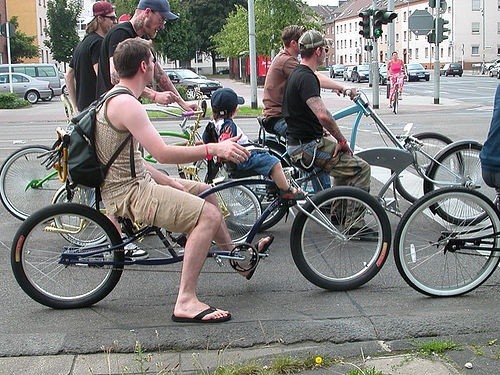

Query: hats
297 29 333 51
119 13 133 23
211 87 245 110
92 2 114 16
137 0 179 22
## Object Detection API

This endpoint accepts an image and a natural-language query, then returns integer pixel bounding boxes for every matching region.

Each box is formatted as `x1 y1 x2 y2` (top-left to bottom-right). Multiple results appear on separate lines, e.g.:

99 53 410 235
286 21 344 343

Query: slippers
245 234 274 281
172 305 231 323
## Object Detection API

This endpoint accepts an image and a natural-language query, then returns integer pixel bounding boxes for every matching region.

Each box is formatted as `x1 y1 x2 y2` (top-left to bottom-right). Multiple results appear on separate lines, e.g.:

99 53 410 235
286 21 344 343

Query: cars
343 61 389 85
330 63 345 79
0 73 52 104
403 63 430 82
162 69 223 100
484 58 500 80
58 72 70 96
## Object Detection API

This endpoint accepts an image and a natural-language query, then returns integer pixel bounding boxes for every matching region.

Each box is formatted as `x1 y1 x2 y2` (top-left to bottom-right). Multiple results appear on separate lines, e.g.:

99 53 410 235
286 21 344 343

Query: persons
64 1 119 118
282 29 380 238
262 24 358 211
478 83 500 189
387 51 408 108
95 0 202 261
94 37 275 323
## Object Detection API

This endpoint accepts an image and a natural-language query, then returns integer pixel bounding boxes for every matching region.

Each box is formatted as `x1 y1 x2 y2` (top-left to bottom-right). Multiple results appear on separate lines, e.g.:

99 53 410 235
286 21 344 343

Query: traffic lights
358 9 371 40
427 33 436 44
438 18 449 44
373 10 384 38
382 10 397 25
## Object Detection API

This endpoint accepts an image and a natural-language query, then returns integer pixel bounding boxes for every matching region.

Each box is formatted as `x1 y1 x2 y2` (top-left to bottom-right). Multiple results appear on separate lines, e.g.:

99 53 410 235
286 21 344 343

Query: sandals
279 185 308 200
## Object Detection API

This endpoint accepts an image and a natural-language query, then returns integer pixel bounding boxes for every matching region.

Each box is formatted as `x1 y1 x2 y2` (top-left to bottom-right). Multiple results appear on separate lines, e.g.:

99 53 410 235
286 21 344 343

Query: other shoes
331 214 346 225
398 96 402 100
345 225 378 242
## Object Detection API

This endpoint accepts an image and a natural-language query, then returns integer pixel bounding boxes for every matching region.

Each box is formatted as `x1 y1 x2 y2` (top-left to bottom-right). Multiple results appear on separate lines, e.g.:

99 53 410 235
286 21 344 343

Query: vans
0 63 63 102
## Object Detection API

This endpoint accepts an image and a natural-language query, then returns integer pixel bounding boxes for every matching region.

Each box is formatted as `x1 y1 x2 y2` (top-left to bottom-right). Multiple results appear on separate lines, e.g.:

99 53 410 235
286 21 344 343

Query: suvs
440 63 464 78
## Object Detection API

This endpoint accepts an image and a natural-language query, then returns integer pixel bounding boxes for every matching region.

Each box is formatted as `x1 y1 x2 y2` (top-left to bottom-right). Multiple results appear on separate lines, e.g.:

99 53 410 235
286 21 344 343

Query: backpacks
203 118 232 168
64 88 137 187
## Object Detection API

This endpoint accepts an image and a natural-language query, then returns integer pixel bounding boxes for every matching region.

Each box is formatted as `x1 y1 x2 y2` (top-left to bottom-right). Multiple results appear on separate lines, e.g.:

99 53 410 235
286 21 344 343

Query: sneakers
107 235 150 259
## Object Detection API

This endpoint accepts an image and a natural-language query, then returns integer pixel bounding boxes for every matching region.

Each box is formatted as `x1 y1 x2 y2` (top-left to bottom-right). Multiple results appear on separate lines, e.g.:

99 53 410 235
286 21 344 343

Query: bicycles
1 86 500 311
388 74 407 114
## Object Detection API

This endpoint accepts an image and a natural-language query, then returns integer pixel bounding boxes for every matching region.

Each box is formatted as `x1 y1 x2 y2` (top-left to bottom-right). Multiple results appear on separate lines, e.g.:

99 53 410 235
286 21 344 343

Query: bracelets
338 139 347 145
205 146 212 161
151 92 157 101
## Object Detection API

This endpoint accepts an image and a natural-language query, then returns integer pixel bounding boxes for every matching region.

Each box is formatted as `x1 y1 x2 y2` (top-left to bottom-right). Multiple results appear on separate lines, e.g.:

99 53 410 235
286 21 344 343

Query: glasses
150 9 168 24
102 14 118 22
320 45 329 53
